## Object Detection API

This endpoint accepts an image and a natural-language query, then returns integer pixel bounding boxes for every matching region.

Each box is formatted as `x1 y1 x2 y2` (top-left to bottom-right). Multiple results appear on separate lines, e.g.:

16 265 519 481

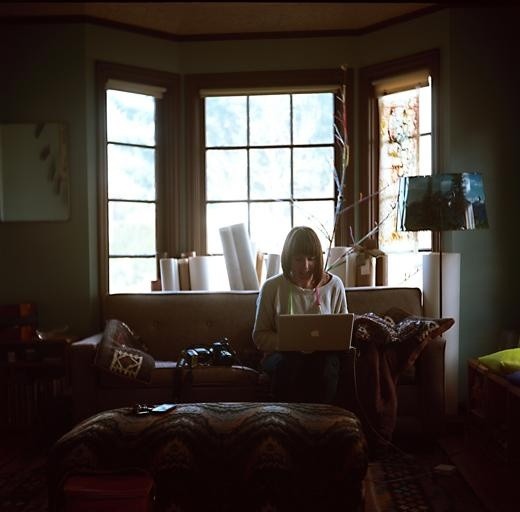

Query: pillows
478 348 520 375
382 307 455 385
504 371 520 386
94 318 155 387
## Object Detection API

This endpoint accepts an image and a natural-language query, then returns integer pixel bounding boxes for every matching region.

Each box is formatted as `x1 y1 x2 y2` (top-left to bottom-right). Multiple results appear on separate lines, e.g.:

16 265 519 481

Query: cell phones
151 403 176 413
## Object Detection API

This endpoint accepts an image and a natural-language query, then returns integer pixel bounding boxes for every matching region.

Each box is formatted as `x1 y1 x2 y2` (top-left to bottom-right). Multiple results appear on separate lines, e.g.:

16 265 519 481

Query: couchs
69 288 447 440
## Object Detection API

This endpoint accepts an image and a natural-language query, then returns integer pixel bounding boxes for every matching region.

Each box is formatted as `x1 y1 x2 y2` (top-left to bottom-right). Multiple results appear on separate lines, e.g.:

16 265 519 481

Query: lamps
401 171 490 337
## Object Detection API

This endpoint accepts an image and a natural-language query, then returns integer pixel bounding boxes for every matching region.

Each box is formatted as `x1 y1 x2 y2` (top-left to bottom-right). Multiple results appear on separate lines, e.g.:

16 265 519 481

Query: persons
254 225 349 403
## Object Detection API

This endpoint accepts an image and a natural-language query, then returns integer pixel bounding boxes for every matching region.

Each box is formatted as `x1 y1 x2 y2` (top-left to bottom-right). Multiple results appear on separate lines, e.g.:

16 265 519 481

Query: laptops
278 313 354 351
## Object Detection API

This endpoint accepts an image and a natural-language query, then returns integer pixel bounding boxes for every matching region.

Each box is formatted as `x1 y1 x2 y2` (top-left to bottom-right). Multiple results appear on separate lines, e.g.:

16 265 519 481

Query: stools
63 474 155 512
